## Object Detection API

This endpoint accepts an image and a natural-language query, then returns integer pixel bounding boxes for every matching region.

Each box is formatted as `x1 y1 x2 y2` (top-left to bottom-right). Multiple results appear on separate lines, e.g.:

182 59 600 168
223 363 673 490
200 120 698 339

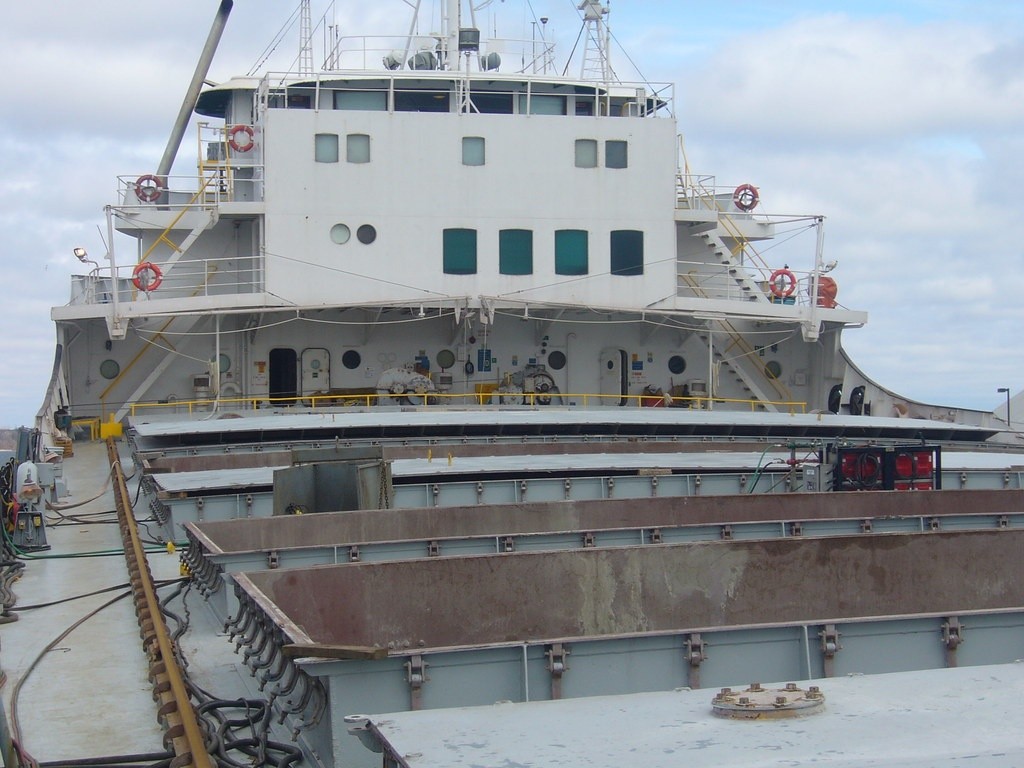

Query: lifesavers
769 269 796 297
135 175 163 202
228 125 254 153
733 183 759 211
132 263 163 291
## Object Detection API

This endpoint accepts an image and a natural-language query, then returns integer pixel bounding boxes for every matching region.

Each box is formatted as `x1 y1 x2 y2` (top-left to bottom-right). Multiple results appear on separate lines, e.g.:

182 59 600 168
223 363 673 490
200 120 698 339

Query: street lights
998 388 1011 427
